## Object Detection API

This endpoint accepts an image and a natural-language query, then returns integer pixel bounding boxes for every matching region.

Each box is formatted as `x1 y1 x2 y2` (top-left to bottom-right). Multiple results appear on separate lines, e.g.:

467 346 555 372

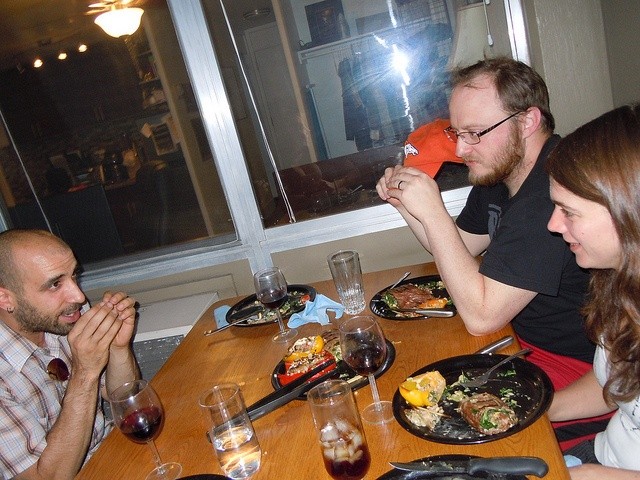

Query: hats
403 118 465 179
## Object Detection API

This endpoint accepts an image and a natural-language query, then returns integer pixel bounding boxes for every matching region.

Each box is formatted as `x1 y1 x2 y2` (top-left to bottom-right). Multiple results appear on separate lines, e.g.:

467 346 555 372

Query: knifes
394 309 456 319
389 457 548 478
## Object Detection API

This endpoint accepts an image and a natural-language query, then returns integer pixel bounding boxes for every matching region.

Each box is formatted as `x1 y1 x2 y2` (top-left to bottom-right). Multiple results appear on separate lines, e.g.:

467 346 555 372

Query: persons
376 46 599 392
547 102 639 480
0 229 141 480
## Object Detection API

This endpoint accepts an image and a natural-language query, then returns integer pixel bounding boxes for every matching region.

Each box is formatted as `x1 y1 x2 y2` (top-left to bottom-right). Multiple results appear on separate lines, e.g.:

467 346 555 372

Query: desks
98 142 139 192
75 254 571 479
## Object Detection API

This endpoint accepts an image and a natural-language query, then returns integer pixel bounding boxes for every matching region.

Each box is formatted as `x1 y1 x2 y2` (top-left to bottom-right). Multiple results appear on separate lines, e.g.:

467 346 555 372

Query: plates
370 275 457 320
392 354 556 445
225 285 316 327
377 455 529 480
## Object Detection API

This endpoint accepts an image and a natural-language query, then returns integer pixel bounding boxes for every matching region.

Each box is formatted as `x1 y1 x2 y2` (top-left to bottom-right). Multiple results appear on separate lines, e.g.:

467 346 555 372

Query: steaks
390 283 435 310
459 391 518 436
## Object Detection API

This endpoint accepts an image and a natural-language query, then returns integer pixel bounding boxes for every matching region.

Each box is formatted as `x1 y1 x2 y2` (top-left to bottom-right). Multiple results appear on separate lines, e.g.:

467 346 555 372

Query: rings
398 180 404 190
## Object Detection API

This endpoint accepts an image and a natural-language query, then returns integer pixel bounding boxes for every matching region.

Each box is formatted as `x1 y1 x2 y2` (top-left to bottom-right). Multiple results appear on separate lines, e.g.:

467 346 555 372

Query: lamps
84 0 145 41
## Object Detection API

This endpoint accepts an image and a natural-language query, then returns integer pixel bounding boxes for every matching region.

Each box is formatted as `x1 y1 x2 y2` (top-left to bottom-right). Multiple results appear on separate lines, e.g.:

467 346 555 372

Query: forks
467 347 531 388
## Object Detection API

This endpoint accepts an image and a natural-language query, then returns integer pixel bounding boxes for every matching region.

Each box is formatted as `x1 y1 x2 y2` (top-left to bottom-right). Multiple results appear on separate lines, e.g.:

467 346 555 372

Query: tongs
206 359 348 443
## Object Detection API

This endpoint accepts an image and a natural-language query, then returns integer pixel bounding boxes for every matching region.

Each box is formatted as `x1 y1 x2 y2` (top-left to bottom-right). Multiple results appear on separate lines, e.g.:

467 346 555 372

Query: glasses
43 356 71 384
442 110 524 146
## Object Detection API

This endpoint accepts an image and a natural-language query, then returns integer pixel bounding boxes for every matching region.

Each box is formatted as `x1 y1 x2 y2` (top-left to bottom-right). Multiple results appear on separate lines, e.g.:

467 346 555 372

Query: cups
326 249 368 316
199 383 262 479
307 379 371 479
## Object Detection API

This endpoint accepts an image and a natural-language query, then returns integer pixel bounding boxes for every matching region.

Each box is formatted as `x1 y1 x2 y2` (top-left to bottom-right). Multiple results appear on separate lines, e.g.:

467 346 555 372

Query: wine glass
109 377 182 480
253 266 298 343
340 315 395 425
